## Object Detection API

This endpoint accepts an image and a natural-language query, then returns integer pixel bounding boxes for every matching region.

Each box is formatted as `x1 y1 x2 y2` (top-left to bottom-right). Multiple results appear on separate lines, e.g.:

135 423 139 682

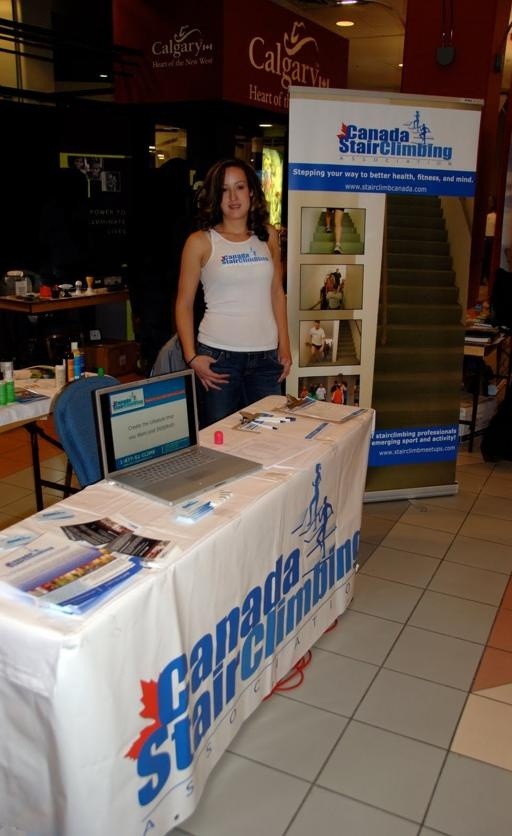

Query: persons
298 207 360 407
175 158 294 430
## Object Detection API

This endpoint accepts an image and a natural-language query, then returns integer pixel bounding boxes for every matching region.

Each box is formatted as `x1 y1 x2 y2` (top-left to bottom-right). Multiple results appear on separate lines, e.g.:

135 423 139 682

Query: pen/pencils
250 419 277 431
254 415 297 424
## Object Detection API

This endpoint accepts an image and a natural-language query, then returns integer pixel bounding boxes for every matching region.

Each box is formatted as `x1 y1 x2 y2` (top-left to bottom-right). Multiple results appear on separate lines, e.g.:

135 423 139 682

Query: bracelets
186 353 198 365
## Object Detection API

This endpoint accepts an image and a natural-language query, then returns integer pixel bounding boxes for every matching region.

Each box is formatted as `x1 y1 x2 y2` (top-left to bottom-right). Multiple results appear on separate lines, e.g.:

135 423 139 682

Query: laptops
91 369 263 506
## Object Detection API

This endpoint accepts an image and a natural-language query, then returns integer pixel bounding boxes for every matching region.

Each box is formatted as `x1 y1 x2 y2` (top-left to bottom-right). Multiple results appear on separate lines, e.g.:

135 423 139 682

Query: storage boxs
83 340 137 378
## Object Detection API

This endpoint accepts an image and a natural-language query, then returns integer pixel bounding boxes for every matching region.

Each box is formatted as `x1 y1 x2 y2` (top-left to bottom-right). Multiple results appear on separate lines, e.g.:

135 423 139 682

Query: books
282 395 369 422
463 322 501 358
237 411 330 439
0 516 176 619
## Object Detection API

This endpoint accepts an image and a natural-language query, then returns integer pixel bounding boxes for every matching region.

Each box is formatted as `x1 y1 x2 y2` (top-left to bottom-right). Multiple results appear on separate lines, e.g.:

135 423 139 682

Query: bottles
4 270 27 299
0 368 15 408
65 345 86 384
